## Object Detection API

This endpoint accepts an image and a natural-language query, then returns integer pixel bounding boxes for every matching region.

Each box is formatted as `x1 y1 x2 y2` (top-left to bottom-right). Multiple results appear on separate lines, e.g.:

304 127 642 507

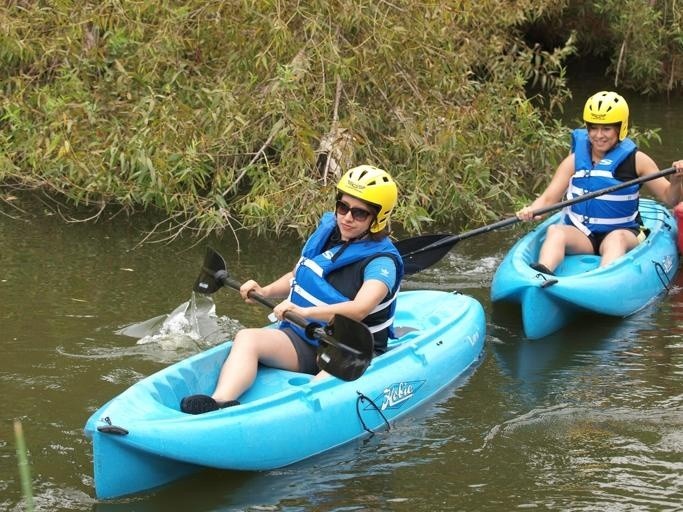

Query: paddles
192 246 374 382
392 167 678 276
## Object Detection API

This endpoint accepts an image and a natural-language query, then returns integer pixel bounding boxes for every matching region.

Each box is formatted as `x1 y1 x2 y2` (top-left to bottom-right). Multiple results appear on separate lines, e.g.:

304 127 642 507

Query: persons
515 91 683 278
179 164 405 414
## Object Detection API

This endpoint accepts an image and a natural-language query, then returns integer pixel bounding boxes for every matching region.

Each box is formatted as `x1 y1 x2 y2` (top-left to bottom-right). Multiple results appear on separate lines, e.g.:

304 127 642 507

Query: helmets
583 90 629 142
336 165 398 235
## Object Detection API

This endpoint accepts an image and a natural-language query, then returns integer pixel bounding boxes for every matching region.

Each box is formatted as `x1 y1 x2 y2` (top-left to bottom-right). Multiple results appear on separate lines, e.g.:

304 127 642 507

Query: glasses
335 200 375 222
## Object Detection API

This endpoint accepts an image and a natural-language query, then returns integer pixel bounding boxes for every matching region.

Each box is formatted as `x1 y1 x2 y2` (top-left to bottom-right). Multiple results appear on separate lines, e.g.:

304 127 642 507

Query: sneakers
180 395 240 414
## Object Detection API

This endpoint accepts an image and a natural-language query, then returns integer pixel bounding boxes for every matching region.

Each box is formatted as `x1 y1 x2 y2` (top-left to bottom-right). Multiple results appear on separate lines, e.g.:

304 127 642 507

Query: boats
490 198 679 342
82 290 486 499
